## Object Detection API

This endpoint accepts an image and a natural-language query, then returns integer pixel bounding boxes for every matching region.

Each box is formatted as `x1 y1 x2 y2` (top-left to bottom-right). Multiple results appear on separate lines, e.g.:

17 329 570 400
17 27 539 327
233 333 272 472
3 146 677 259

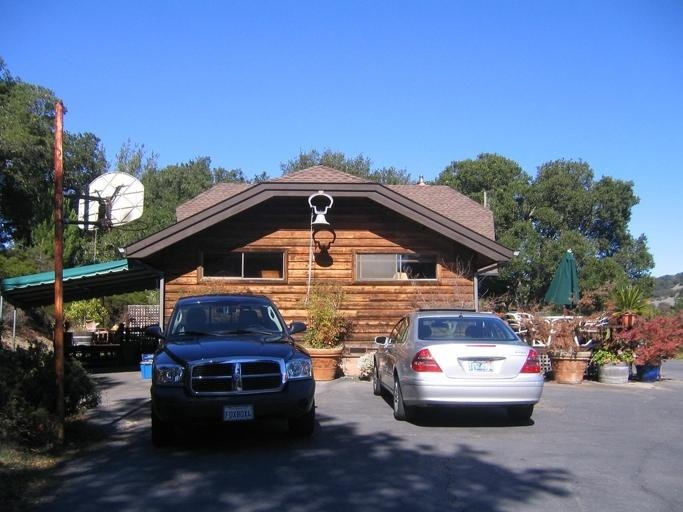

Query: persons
112 323 125 343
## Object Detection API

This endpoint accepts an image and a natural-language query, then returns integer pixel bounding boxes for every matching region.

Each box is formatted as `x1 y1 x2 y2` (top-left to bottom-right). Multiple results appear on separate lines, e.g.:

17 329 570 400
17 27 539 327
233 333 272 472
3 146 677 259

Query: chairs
179 306 265 333
421 324 432 337
465 326 491 337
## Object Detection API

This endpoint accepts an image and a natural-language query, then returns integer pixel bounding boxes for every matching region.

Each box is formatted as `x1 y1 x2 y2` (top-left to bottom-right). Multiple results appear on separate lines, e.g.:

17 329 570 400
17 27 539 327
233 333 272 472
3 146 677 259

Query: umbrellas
542 248 578 312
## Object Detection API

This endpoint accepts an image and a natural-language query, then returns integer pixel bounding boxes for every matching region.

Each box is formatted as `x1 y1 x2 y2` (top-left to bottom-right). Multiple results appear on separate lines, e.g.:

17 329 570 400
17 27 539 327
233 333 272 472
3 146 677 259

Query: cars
369 304 544 427
142 291 315 441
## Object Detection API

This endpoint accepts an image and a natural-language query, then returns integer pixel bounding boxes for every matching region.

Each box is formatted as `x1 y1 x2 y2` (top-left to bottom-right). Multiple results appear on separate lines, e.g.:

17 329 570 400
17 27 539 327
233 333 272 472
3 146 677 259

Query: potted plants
296 279 353 381
547 281 683 386
